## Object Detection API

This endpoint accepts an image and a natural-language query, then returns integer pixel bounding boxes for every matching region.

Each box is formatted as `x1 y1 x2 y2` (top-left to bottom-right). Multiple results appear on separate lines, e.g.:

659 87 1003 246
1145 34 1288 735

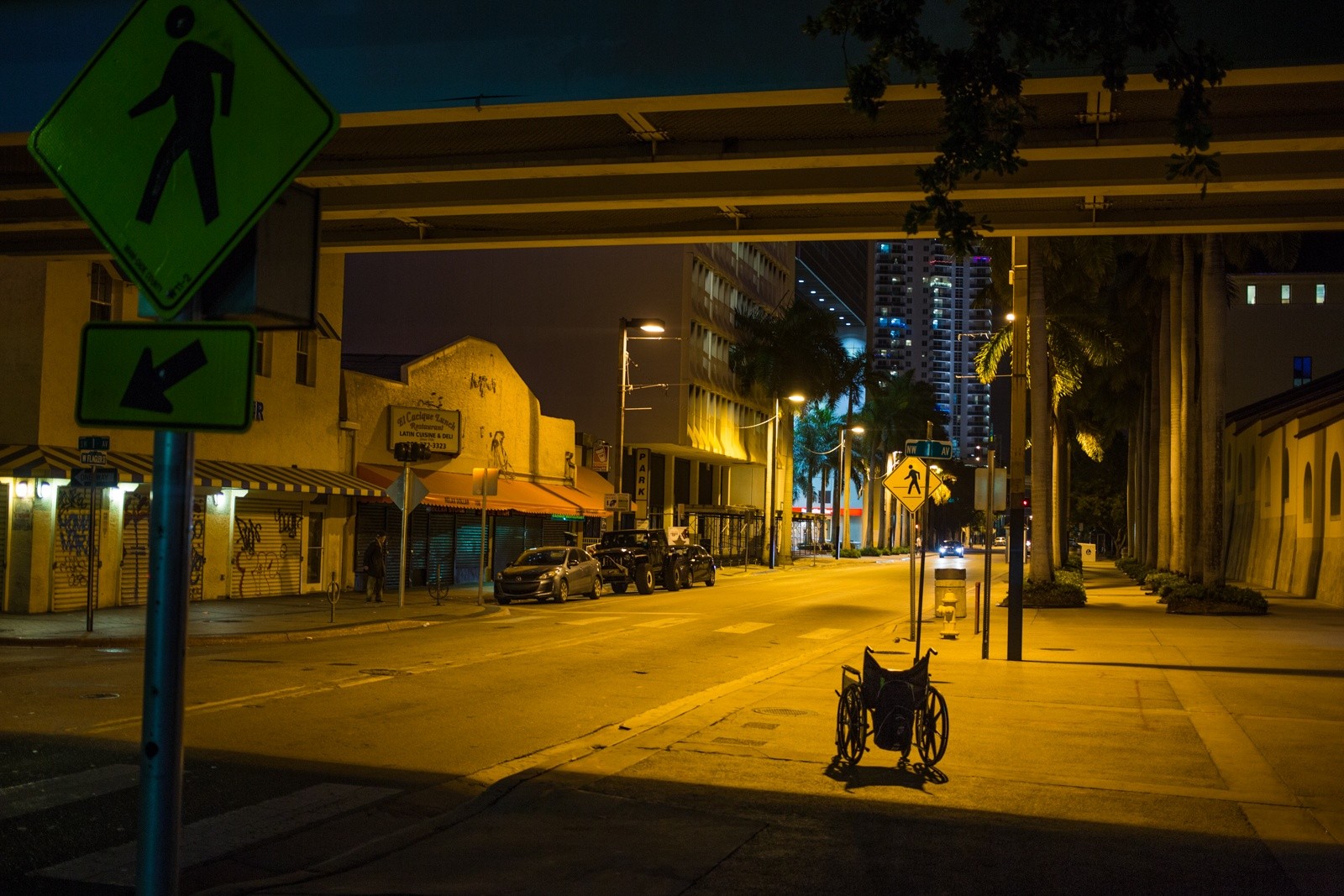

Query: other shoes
366 597 372 602
375 598 383 602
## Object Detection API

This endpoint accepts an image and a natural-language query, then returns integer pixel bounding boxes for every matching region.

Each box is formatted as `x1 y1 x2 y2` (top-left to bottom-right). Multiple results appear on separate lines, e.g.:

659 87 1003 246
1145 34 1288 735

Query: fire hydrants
935 591 959 640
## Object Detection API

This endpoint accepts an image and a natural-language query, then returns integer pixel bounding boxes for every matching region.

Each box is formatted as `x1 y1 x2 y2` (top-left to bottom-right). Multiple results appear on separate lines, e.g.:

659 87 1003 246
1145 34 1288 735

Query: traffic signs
70 467 119 489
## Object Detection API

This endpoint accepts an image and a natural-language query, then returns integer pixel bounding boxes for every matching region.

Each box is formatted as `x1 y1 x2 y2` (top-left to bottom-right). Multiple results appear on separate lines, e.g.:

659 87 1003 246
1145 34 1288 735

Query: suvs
592 528 680 595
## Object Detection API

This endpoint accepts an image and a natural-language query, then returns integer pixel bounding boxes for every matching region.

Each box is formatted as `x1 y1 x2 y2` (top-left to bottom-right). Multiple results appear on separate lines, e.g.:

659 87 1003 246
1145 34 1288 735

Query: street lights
768 392 805 569
614 317 666 534
834 426 864 559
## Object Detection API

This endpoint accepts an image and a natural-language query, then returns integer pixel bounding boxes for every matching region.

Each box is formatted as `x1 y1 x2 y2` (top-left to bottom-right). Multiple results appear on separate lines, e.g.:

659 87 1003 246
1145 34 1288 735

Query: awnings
356 461 641 518
0 444 387 497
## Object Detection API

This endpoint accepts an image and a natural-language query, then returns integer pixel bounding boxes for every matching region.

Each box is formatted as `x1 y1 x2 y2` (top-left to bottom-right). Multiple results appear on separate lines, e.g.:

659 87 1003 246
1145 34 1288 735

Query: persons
916 535 922 555
363 529 387 603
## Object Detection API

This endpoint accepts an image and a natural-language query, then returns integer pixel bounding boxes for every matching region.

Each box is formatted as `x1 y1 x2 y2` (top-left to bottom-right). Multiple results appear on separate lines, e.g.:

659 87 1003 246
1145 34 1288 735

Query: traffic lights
1023 501 1028 506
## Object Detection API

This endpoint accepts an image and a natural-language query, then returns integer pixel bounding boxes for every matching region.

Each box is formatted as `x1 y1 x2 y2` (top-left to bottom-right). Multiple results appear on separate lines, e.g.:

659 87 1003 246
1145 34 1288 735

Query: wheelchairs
835 645 950 766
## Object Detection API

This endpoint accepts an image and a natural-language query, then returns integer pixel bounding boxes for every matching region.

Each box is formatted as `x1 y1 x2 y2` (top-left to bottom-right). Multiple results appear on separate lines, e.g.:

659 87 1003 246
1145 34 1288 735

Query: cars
671 545 716 587
492 547 604 605
995 537 1006 546
1026 541 1030 552
936 541 967 560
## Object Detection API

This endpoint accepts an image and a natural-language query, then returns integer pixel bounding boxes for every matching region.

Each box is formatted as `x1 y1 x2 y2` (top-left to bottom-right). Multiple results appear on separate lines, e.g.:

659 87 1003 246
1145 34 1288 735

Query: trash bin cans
935 568 967 618
